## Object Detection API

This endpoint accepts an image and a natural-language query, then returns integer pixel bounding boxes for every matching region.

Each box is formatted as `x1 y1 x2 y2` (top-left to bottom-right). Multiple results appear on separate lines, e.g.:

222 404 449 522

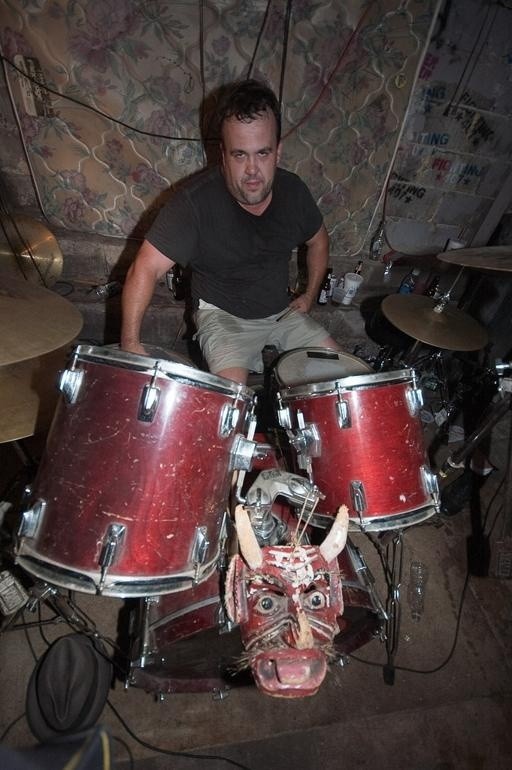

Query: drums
13 344 259 598
268 348 376 407
102 342 198 372
277 368 441 532
124 433 387 700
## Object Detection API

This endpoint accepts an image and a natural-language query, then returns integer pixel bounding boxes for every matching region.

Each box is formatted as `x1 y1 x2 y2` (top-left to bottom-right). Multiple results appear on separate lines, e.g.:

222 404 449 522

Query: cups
446 238 467 252
332 272 363 307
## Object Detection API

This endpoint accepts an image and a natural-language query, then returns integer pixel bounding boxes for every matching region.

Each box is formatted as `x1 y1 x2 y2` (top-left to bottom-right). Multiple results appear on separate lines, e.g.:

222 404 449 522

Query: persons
117 76 347 390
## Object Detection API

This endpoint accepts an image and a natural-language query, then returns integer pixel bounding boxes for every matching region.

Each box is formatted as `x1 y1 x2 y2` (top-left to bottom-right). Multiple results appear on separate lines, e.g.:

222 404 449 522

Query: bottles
369 228 385 260
167 264 185 301
83 281 119 302
400 267 420 294
407 561 428 625
318 260 363 307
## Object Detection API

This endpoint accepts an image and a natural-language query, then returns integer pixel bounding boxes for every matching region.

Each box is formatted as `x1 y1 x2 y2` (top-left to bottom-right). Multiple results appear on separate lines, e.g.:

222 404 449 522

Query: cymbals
1 213 63 287
0 283 83 365
2 340 92 444
436 244 511 272
381 293 489 351
